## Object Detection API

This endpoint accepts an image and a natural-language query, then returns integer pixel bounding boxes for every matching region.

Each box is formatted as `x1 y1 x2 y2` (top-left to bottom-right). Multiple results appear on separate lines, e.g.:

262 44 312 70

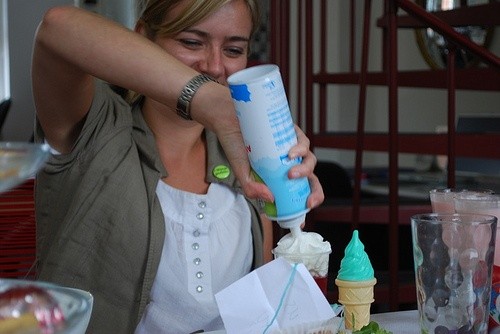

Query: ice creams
336 230 376 330
272 230 332 298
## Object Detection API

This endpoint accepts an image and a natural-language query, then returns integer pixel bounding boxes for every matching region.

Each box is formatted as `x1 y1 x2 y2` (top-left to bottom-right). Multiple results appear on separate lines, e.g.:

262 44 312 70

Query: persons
30 0 324 334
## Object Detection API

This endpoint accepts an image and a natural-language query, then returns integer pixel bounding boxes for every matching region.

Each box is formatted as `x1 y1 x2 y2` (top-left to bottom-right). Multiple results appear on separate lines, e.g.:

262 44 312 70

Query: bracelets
176 74 219 120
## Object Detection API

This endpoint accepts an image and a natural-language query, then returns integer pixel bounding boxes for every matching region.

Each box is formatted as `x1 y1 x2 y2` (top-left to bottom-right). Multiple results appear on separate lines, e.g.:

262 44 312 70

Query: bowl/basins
0 279 94 334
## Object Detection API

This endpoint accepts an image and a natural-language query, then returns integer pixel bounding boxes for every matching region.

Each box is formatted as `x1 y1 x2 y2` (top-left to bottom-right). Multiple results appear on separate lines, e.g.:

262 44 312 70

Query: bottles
227 64 313 237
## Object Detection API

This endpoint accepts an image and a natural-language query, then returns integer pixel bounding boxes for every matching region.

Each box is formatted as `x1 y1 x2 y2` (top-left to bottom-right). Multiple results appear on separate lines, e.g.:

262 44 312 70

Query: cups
271 247 330 298
429 187 500 267
410 213 497 334
0 142 49 192
